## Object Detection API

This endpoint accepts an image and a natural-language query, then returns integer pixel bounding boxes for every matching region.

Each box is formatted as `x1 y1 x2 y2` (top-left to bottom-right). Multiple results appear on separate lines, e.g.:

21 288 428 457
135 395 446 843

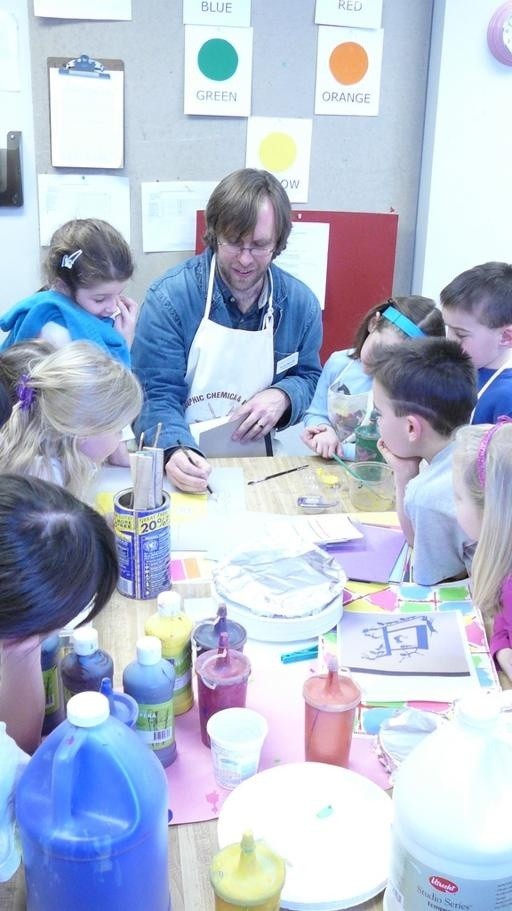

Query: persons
0 475 120 884
130 168 322 493
454 424 512 682
0 341 54 424
299 296 443 463
0 217 138 467
441 261 511 423
0 340 145 485
364 336 478 587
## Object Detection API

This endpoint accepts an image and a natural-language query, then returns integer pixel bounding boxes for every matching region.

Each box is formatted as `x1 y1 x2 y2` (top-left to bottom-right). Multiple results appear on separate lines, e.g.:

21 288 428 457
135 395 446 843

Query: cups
204 708 268 791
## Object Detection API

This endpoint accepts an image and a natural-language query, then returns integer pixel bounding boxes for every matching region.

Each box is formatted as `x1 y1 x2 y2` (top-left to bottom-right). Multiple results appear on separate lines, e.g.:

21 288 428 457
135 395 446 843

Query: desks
74 454 510 910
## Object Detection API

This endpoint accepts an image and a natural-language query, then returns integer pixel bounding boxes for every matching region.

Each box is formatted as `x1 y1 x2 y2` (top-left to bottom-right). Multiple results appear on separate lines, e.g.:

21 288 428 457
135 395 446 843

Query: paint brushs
247 464 308 485
177 439 213 493
152 422 164 447
128 432 145 510
332 453 386 501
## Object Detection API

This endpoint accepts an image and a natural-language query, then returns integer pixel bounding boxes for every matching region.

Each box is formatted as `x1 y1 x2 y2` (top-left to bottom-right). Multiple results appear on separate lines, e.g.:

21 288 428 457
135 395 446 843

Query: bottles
208 831 284 911
60 626 115 717
193 604 253 749
353 414 386 481
34 632 62 735
303 653 361 769
123 587 192 771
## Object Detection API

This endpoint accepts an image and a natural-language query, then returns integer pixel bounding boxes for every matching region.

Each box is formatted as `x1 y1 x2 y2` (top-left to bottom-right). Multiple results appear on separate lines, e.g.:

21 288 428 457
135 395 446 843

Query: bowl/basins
346 461 397 512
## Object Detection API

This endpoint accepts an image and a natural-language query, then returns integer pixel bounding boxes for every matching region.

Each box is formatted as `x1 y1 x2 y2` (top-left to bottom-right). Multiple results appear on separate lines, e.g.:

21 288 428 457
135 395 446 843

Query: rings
257 422 265 429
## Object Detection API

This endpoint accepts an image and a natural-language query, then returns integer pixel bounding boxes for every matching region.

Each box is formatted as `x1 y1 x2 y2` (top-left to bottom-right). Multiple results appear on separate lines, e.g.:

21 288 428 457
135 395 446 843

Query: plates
211 763 393 910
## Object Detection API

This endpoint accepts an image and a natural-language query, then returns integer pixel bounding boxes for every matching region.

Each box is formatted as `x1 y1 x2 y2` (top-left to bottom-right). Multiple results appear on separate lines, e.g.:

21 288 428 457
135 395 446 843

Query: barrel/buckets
14 689 172 911
380 689 512 911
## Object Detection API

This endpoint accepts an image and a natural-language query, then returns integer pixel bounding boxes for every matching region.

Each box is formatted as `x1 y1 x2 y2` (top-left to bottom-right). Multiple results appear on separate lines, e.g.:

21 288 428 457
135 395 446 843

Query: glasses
216 235 277 257
